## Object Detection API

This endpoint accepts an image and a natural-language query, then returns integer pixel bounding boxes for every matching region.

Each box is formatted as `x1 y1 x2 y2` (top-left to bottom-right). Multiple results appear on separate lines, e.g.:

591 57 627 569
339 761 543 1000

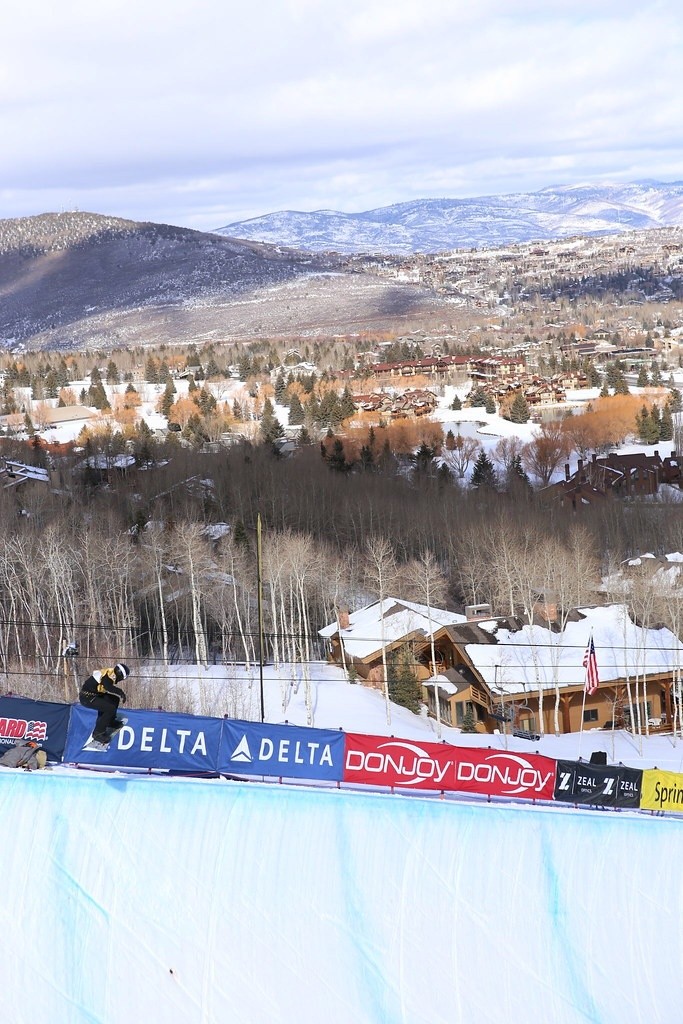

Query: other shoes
92 732 110 742
110 721 123 728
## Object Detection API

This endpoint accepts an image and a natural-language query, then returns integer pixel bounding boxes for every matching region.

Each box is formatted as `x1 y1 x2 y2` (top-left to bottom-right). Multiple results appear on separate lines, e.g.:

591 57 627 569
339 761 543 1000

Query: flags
583 636 599 695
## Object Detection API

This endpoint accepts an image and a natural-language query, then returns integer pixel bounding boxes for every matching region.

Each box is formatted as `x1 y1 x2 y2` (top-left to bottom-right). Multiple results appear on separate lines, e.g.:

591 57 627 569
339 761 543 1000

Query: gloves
121 694 126 703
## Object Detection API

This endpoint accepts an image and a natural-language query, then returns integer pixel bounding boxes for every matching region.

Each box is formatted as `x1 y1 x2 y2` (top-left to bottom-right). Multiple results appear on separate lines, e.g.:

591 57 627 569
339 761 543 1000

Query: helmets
114 663 129 680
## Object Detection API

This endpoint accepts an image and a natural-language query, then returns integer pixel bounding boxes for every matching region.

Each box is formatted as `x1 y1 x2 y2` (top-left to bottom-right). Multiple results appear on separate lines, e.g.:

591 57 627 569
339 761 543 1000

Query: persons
79 663 130 743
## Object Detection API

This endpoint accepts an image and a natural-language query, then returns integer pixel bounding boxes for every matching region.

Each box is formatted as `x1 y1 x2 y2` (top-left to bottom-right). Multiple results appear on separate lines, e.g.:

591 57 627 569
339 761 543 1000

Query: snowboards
92 718 128 750
